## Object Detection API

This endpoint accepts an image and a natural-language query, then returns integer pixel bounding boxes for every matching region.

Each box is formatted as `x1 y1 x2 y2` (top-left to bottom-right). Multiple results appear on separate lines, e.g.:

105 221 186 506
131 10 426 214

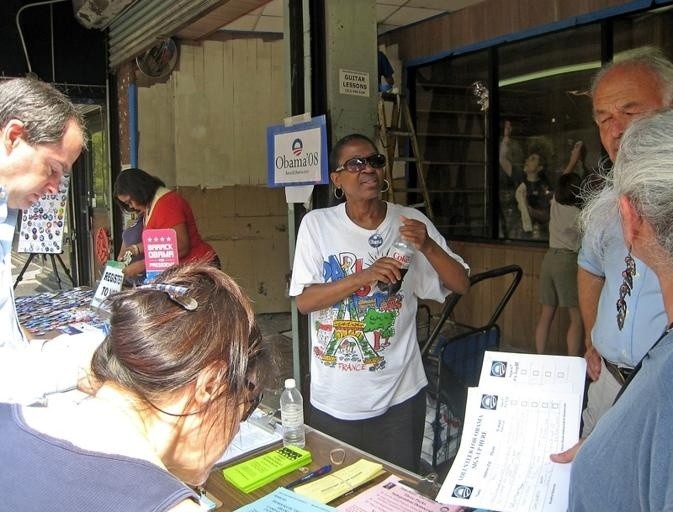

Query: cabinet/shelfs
406 80 491 235
417 264 525 473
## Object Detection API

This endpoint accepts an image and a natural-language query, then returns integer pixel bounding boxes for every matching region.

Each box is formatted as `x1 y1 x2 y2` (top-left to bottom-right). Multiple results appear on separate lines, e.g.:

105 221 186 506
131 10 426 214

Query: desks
202 431 443 512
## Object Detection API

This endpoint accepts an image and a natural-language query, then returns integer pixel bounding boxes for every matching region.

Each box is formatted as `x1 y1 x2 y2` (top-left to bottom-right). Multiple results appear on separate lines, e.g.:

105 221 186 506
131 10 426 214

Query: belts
605 357 634 387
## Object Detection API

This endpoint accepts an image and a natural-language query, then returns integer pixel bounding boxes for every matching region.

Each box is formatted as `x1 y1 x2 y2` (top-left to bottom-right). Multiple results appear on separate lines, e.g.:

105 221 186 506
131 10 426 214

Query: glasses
239 377 261 419
335 154 386 173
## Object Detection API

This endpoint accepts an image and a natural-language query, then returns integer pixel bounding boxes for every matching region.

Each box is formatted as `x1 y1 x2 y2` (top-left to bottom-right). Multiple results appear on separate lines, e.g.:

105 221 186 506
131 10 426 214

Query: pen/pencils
285 464 333 489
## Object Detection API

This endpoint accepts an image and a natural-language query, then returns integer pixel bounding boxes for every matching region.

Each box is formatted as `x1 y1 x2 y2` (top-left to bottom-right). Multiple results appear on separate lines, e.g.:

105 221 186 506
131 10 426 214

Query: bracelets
130 244 140 255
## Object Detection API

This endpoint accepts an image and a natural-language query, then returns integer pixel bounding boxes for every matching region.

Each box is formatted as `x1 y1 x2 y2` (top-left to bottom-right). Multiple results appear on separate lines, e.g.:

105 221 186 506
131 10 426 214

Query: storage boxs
419 394 462 467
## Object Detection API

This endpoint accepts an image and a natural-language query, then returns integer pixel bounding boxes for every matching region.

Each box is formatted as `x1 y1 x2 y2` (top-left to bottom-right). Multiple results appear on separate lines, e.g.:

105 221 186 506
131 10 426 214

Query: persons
112 169 220 281
548 110 671 510
114 208 146 293
500 120 553 242
533 140 588 355
1 241 268 510
570 43 673 446
0 70 109 403
286 132 470 477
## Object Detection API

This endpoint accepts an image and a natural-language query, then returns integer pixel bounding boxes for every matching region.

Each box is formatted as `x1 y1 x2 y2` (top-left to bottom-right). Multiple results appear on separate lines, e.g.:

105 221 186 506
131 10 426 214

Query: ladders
378 90 435 225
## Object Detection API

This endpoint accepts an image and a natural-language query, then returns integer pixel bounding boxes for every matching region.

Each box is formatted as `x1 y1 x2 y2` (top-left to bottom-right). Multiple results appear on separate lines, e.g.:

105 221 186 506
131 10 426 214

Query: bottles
279 378 306 450
378 235 416 296
